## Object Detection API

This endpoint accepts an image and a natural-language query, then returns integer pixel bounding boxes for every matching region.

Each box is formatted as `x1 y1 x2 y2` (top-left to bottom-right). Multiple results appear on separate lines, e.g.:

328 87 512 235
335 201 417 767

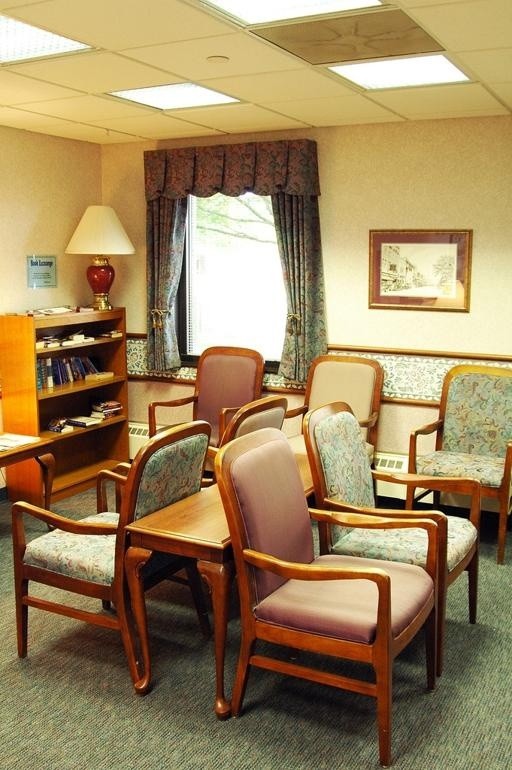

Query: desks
124 455 314 717
0 432 57 536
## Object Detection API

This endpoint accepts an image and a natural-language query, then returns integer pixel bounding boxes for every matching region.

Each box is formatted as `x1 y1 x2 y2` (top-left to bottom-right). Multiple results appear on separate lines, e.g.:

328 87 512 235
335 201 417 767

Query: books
49 400 123 434
0 433 41 451
68 306 94 313
36 329 127 350
37 306 73 315
35 356 113 390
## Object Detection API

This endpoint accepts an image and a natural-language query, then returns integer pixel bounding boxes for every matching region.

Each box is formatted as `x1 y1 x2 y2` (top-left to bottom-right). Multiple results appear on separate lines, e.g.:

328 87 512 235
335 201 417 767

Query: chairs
10 421 214 694
303 401 478 674
112 396 289 492
407 366 512 564
148 347 265 446
214 427 439 767
282 354 383 470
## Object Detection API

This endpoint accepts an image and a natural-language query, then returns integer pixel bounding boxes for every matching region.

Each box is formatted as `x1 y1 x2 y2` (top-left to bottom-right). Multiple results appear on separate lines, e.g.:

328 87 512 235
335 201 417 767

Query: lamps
64 206 135 313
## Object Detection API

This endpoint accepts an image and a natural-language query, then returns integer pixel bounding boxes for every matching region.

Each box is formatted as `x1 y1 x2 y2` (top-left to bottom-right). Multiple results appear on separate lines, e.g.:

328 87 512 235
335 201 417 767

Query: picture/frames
367 229 474 312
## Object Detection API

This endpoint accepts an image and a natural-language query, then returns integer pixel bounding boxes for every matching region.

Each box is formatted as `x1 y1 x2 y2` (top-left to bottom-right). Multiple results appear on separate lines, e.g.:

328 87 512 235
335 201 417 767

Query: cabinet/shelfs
0 306 132 506
